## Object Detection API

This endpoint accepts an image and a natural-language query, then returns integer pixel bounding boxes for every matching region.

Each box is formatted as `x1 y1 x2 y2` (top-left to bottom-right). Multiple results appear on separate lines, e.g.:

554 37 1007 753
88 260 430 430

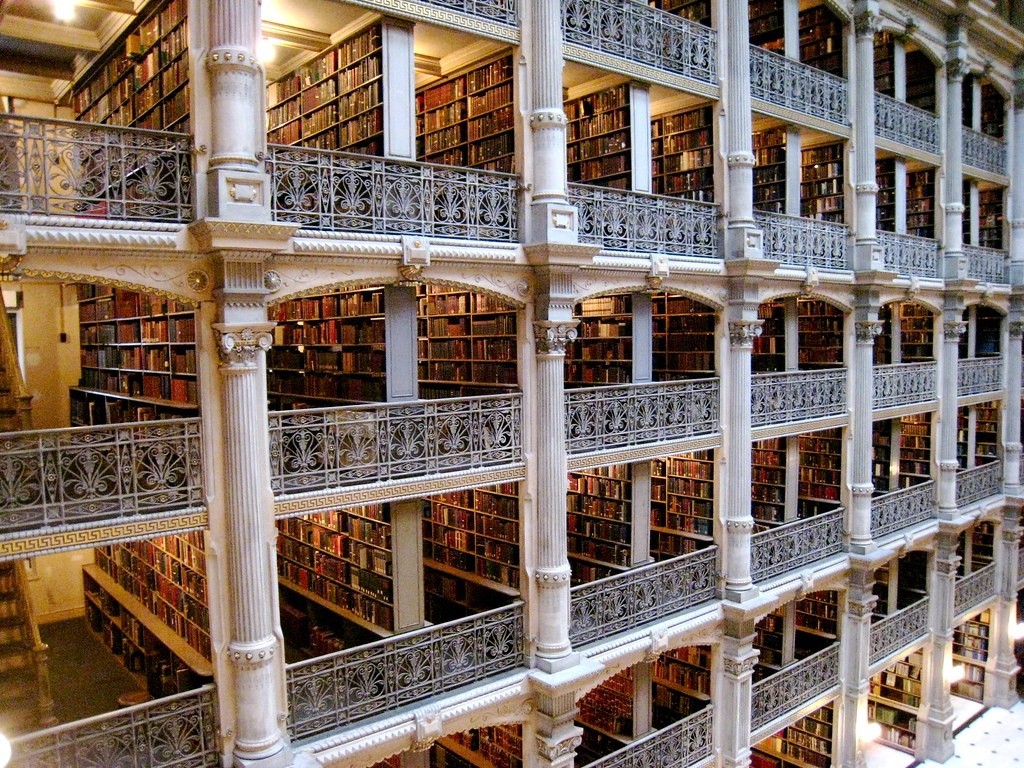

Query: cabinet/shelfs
68 0 1009 768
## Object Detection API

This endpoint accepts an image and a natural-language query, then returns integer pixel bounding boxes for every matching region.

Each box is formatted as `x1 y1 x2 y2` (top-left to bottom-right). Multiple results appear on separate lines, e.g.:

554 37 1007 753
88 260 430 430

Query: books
649 106 714 255
562 84 631 243
83 529 216 736
279 479 523 696
268 282 518 456
266 23 383 216
563 292 716 440
415 55 515 241
372 722 523 768
574 642 712 768
649 0 1005 138
573 451 718 630
751 124 1003 284
67 280 202 492
751 295 1006 768
74 0 195 219
